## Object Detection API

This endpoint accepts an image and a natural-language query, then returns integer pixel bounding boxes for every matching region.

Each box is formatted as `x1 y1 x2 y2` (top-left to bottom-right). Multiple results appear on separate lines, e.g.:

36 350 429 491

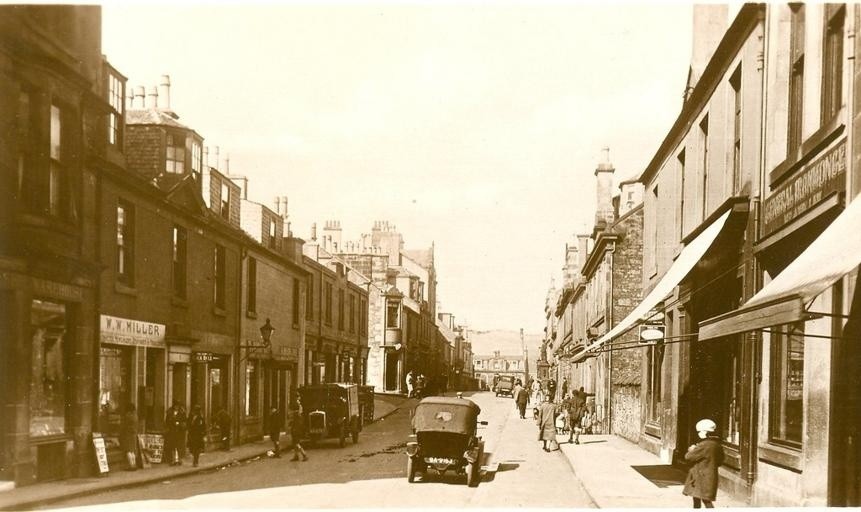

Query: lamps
232 313 274 351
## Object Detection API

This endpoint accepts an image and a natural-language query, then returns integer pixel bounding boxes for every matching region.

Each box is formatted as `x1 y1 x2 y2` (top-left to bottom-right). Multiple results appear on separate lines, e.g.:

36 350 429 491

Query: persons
683 419 725 507
290 410 308 462
218 406 231 450
119 403 138 469
165 399 205 468
267 408 282 459
511 375 595 451
405 370 448 398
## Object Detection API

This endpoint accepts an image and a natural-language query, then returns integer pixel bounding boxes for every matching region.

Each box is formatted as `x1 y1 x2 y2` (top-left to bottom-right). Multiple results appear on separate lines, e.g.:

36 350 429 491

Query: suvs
295 380 364 448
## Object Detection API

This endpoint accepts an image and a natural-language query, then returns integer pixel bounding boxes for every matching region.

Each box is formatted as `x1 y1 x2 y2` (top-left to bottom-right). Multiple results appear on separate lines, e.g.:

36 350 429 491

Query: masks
697 430 708 440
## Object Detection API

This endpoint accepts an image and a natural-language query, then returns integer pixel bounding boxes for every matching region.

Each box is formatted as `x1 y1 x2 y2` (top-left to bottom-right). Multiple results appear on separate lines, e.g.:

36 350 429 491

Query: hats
695 418 716 432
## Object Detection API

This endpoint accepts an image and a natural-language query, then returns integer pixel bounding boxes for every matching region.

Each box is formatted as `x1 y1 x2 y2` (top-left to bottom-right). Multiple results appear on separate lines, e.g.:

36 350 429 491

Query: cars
495 375 517 398
406 395 489 487
